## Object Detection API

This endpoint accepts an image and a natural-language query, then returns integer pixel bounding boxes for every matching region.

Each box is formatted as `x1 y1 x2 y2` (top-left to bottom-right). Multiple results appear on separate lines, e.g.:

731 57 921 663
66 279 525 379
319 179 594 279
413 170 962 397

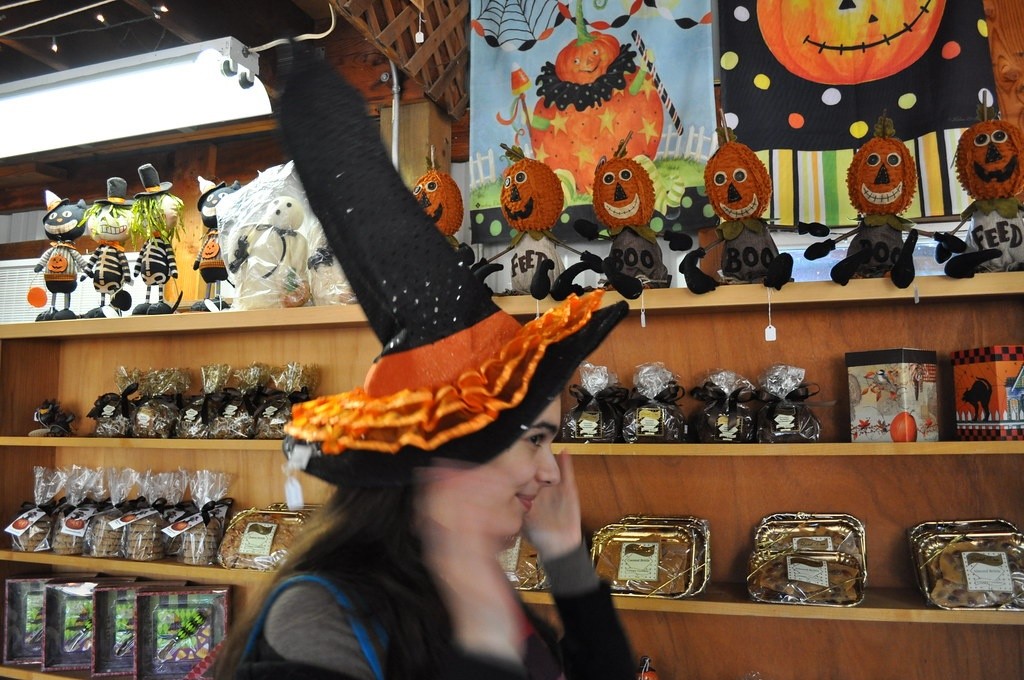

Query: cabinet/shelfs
2 269 1024 680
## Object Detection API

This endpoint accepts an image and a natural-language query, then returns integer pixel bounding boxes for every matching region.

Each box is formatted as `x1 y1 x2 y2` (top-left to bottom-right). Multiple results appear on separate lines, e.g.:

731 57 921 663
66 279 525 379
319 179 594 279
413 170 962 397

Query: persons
219 298 644 680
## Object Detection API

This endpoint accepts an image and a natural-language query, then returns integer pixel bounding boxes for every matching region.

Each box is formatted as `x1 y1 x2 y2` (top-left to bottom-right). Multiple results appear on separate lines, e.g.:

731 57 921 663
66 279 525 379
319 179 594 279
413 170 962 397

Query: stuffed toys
309 232 357 304
469 141 602 302
188 174 244 310
679 107 829 295
231 192 307 307
128 162 187 314
31 188 93 321
551 130 692 305
410 144 476 267
80 176 133 318
939 89 1024 280
805 104 968 290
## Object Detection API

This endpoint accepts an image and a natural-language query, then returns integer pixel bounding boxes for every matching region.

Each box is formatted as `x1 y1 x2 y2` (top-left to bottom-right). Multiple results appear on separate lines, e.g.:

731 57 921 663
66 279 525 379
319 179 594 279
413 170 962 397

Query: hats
275 50 630 486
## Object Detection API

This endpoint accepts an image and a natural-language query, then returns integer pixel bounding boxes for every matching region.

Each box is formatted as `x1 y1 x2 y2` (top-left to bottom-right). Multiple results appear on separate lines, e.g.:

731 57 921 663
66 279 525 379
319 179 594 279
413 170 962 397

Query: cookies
15 510 219 565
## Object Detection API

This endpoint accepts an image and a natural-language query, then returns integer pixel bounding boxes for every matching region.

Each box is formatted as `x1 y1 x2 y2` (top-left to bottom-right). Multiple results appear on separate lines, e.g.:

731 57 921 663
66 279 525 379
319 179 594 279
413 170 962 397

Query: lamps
188 38 262 102
